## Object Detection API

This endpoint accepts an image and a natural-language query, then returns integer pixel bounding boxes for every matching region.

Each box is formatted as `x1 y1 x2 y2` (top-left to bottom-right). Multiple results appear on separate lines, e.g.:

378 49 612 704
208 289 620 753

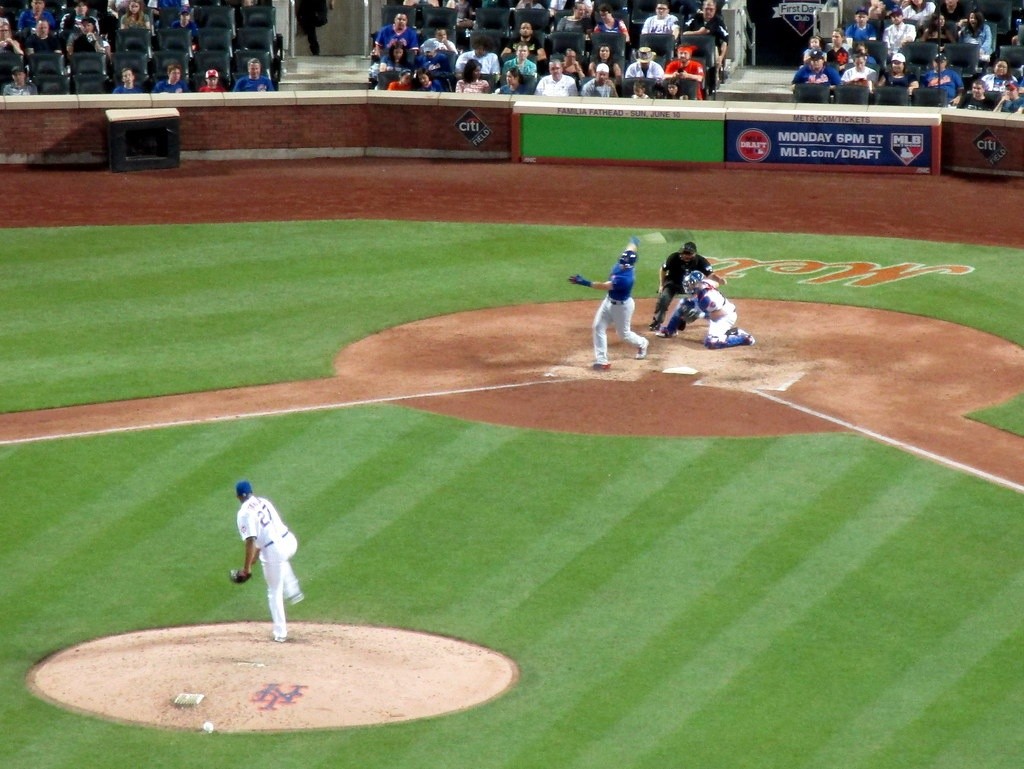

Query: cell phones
859 78 864 80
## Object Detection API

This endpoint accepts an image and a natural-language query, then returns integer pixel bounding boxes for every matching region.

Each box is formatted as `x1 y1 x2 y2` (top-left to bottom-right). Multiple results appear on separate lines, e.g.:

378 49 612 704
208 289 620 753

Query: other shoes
649 322 660 331
288 592 304 605
742 333 755 346
655 327 678 338
275 636 287 642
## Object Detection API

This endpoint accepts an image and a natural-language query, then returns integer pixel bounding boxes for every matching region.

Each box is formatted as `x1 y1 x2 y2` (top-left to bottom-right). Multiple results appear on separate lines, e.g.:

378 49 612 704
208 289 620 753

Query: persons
231 481 304 642
242 0 258 6
232 58 275 91
0 0 197 95
649 242 727 330
792 0 1024 115
369 0 730 100
655 270 755 349
198 69 227 92
569 236 649 369
295 0 335 56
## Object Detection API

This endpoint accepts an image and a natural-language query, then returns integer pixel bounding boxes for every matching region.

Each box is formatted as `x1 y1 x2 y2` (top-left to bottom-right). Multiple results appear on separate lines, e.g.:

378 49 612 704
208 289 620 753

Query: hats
236 481 251 496
179 6 191 15
81 17 95 25
205 69 219 79
934 53 948 61
596 63 609 72
632 46 656 64
891 52 906 62
855 6 868 15
1006 81 1019 90
12 65 25 74
810 51 824 60
683 241 697 254
889 7 902 16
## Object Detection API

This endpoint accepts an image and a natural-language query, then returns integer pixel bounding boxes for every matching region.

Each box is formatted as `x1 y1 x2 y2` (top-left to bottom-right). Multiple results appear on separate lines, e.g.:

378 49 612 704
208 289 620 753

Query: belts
612 301 623 305
265 532 288 547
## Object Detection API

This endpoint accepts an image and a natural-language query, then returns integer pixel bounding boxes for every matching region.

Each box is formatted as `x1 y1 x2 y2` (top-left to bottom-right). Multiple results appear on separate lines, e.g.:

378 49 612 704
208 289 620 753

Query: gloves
569 274 591 288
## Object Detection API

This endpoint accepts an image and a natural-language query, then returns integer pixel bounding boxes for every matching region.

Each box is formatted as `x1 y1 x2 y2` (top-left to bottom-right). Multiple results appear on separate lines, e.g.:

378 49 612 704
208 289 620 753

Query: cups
810 75 816 83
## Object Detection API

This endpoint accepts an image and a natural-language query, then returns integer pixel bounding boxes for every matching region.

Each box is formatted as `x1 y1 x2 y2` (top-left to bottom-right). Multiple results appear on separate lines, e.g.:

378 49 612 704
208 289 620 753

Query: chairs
792 0 1024 114
0 0 288 98
366 2 734 104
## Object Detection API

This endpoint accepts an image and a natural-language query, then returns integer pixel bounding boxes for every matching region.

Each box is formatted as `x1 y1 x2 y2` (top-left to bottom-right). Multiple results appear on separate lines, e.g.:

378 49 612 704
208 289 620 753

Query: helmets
619 251 637 265
687 270 704 282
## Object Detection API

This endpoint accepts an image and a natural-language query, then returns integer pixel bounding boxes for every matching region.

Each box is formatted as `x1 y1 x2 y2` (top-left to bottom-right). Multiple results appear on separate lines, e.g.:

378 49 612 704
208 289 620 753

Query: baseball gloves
678 304 697 324
229 569 252 584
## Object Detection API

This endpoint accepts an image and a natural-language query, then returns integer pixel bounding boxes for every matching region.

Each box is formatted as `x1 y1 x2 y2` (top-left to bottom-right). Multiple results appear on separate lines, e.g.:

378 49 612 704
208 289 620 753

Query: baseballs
203 722 214 732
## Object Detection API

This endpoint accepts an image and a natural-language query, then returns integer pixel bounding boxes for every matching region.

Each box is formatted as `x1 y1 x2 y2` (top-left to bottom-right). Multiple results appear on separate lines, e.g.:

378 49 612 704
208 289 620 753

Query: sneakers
593 361 610 370
636 337 649 359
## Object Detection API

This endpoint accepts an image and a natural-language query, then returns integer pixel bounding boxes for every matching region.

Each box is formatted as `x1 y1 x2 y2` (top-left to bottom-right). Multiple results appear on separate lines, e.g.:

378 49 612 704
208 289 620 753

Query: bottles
87 31 93 42
